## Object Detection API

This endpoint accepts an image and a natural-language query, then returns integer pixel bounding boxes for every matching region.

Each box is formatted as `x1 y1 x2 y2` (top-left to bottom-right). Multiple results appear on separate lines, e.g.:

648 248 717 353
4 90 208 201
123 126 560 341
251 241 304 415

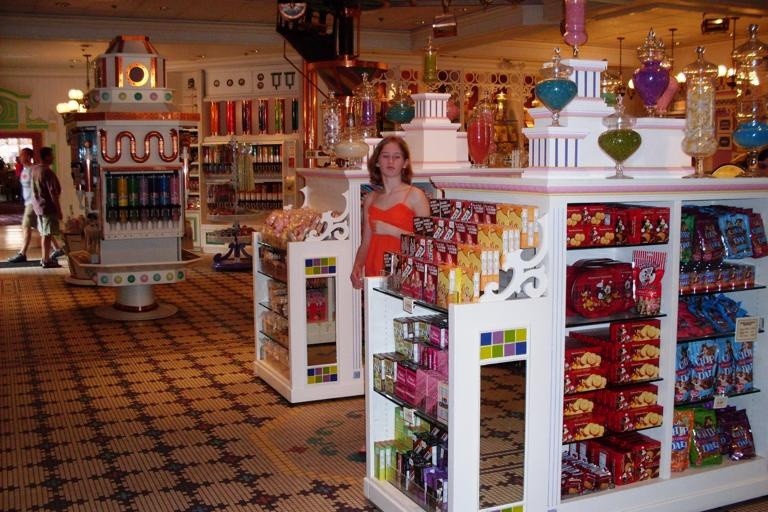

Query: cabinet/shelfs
245 160 365 404
195 65 307 259
359 173 767 512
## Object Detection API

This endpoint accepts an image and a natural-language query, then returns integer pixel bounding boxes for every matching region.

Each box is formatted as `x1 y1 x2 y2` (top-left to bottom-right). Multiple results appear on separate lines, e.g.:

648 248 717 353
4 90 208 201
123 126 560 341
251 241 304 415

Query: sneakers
7 252 26 263
50 249 65 258
42 258 62 268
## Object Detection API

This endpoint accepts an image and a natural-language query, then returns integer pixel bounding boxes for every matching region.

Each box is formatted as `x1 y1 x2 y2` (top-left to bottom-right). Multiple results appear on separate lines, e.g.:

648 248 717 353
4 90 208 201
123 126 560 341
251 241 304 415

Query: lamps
54 59 88 121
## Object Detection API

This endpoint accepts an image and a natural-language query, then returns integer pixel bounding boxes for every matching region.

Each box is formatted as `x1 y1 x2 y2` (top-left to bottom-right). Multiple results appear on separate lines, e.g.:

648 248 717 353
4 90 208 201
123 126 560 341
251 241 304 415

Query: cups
478 109 494 167
467 119 490 169
475 121 490 163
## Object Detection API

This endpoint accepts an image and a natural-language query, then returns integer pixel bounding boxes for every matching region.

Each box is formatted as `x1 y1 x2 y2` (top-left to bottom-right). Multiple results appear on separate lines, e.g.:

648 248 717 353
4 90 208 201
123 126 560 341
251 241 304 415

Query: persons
7 148 65 262
350 136 431 289
31 147 63 268
14 157 23 180
